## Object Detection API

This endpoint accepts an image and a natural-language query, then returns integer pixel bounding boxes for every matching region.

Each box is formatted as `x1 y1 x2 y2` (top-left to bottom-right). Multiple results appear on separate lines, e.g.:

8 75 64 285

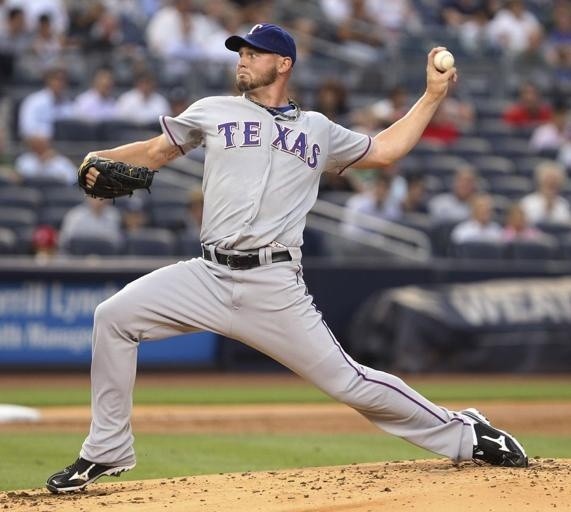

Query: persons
48 23 529 495
1 1 571 253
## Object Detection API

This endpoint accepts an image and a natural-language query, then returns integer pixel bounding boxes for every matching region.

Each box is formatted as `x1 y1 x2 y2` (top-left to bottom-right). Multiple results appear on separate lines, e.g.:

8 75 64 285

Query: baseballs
434 51 454 72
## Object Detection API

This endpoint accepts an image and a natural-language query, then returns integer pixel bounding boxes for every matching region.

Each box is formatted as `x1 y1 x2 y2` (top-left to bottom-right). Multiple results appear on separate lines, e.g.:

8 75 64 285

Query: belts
201 250 292 270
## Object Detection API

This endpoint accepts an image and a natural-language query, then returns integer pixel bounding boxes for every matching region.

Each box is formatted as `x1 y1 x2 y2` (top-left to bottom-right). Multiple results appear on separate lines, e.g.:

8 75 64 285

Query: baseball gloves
78 156 155 202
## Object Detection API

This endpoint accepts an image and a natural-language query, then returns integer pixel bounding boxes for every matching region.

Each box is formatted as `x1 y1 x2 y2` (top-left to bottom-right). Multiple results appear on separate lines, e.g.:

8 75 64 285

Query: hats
225 23 296 63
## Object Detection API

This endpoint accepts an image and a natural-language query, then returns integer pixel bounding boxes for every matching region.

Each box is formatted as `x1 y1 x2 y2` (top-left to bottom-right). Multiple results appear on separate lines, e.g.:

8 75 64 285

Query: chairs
0 27 571 257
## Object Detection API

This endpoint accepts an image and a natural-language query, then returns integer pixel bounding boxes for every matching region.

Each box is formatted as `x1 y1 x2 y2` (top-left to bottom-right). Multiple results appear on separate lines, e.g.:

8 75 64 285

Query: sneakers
458 407 528 467
46 457 137 494
